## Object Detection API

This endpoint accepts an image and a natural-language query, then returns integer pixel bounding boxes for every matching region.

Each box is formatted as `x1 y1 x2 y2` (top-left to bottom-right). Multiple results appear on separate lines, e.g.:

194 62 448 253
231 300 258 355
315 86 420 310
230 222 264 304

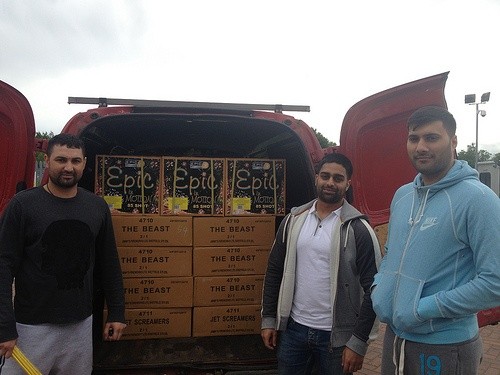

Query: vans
0 69 453 375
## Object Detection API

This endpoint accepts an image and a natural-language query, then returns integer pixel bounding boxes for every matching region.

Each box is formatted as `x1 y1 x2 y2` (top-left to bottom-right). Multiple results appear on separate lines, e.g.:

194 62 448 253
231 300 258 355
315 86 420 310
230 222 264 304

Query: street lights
464 92 490 171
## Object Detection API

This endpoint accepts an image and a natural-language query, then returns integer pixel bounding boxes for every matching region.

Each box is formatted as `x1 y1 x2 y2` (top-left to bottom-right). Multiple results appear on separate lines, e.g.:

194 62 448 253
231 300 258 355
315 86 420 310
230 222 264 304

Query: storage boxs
104 277 194 310
111 216 193 246
160 157 226 215
193 216 274 246
192 245 273 277
95 154 160 216
192 305 261 337
193 275 264 307
102 308 192 341
116 246 193 279
225 158 286 216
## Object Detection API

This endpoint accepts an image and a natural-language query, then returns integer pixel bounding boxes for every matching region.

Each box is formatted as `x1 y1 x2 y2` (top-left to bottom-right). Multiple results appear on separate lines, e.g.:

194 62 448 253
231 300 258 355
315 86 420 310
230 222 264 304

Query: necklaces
47 183 56 197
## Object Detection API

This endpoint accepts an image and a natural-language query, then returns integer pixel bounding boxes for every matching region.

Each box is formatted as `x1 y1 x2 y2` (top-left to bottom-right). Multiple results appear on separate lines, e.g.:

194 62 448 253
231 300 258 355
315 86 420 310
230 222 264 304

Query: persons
0 134 127 375
370 105 500 375
261 153 383 375
